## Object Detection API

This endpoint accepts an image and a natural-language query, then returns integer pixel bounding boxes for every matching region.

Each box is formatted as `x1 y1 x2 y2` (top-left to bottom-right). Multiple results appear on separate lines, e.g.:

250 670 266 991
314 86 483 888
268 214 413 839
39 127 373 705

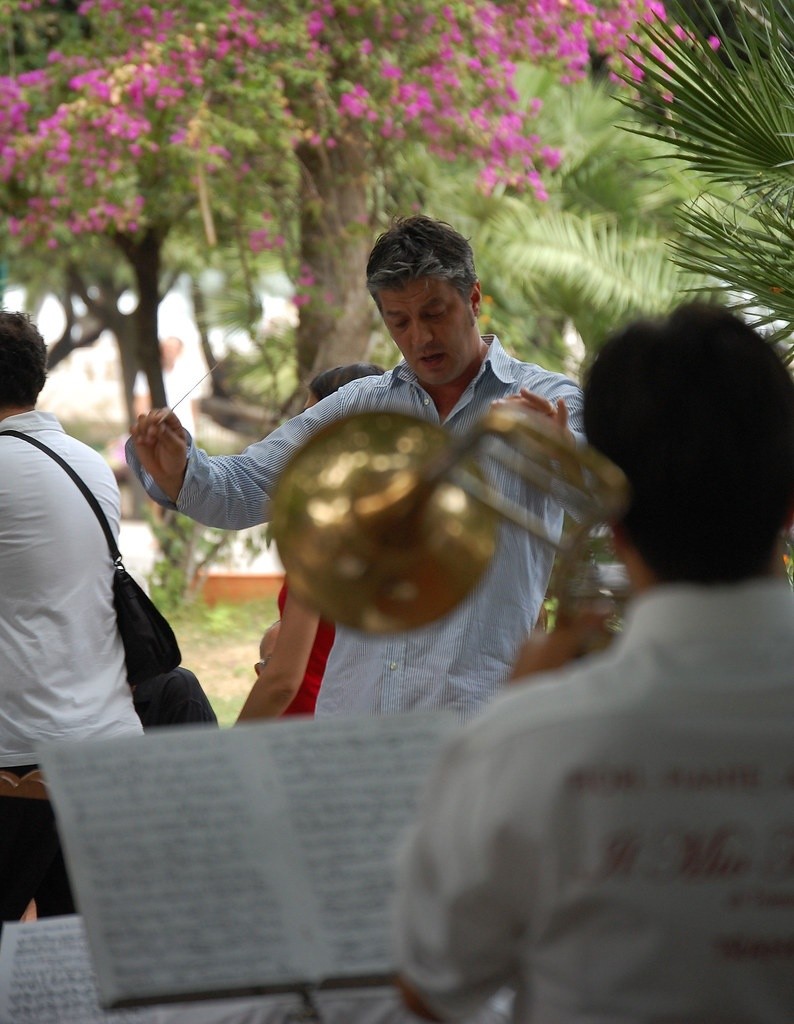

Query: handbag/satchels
111 562 222 728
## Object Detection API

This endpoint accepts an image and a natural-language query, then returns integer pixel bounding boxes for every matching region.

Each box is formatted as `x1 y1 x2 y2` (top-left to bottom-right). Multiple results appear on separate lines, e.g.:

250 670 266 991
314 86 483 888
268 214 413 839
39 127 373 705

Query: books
0 709 512 1024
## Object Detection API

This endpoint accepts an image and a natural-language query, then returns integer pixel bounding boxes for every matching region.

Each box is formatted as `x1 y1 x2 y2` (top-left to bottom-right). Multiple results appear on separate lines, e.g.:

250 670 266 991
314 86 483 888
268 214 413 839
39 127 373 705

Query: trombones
264 396 630 657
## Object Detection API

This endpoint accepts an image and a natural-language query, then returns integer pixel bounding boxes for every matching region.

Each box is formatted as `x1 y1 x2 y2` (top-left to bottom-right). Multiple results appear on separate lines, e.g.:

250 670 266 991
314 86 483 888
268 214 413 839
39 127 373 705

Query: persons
388 300 794 1024
125 213 589 726
0 311 220 947
